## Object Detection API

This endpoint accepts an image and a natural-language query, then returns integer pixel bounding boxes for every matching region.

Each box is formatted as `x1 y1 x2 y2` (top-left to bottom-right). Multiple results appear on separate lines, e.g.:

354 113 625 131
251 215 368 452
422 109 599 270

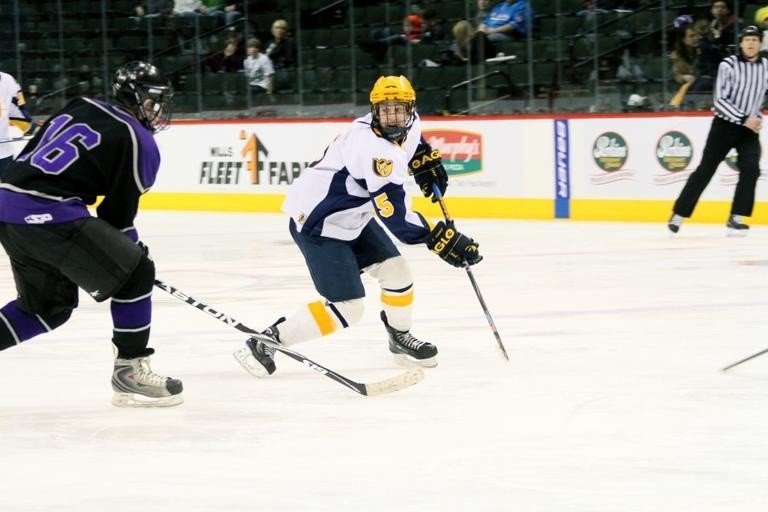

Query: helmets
113 61 174 135
368 74 417 142
739 24 763 40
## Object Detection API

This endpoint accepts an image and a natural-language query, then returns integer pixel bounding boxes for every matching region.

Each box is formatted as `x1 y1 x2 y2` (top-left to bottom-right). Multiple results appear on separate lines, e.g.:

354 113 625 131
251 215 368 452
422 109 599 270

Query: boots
245 316 286 375
111 338 183 398
381 311 438 359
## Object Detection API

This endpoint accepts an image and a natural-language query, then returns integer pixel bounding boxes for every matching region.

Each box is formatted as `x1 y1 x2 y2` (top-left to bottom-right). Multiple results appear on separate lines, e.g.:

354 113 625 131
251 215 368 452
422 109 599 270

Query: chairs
0 1 767 119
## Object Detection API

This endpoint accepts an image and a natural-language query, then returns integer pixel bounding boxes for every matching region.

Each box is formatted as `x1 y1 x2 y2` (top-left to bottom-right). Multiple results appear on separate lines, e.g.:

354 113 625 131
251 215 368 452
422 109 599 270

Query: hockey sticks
154 282 424 395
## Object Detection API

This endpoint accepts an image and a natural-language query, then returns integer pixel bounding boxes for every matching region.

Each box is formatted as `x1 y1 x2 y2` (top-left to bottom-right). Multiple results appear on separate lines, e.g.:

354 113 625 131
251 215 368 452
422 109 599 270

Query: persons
0 61 182 398
0 72 46 177
668 25 768 232
246 74 483 375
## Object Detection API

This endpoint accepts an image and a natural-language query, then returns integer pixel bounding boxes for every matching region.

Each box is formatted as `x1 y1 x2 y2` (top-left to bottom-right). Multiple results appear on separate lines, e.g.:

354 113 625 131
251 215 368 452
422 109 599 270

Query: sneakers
727 214 749 230
669 211 684 232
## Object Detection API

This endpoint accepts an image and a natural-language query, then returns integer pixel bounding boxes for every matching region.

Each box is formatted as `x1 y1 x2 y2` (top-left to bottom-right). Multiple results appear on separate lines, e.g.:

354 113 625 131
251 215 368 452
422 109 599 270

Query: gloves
424 221 483 268
409 143 448 203
